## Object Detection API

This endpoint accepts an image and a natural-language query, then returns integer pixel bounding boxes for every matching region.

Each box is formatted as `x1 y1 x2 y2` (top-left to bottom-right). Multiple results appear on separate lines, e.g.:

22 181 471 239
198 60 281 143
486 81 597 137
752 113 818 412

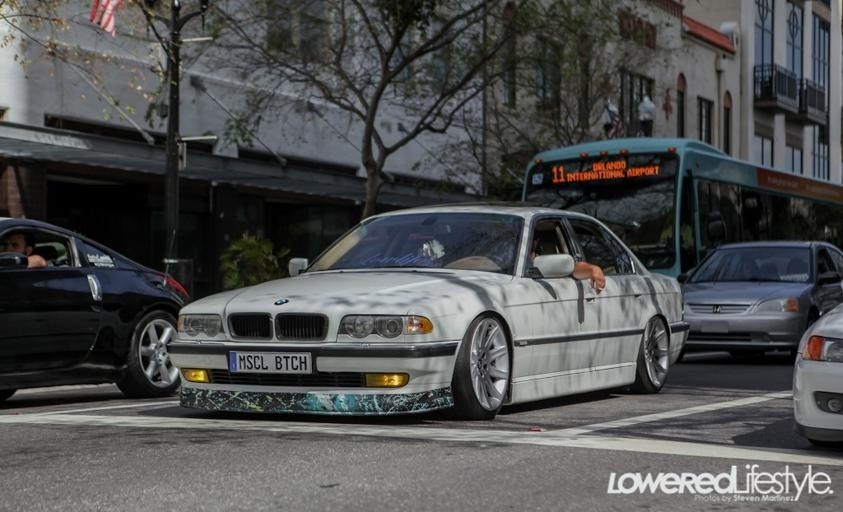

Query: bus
521 137 843 287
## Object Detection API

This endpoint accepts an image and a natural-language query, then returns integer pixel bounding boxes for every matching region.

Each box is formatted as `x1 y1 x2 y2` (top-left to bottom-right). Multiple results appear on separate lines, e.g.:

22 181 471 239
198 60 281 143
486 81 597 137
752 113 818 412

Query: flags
86 1 123 38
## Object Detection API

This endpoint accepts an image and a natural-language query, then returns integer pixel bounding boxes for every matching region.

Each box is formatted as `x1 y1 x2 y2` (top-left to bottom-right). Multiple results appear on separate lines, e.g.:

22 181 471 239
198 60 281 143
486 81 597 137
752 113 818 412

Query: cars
676 240 843 363
164 203 691 420
0 216 191 403
792 302 843 447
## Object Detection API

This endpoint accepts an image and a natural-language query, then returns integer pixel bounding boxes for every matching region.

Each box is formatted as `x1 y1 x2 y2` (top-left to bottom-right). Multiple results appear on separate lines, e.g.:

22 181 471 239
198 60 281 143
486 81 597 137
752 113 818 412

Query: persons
658 207 694 251
592 286 605 291
471 220 606 293
739 258 759 279
601 98 623 139
785 257 807 281
0 229 48 271
638 93 656 138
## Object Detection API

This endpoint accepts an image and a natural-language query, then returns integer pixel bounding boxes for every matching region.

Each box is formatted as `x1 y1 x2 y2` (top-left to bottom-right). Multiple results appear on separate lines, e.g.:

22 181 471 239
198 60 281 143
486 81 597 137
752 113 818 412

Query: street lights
603 95 657 138
